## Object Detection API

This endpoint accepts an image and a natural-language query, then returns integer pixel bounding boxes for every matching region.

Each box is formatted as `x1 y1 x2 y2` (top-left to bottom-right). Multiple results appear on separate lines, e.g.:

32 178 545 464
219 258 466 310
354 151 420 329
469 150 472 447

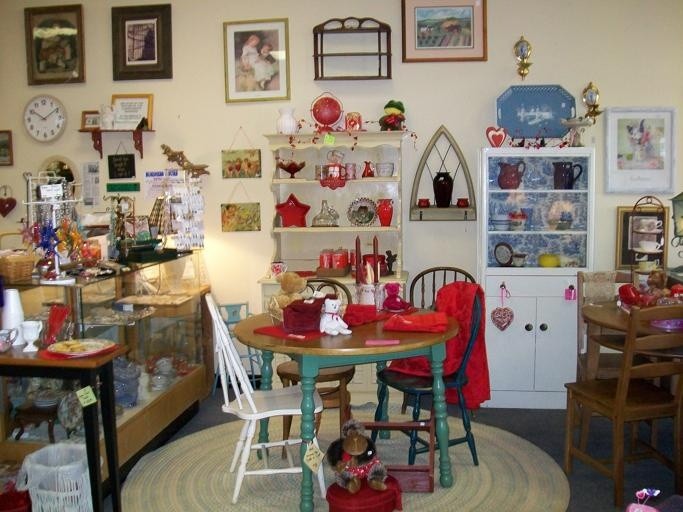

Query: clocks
23 94 67 143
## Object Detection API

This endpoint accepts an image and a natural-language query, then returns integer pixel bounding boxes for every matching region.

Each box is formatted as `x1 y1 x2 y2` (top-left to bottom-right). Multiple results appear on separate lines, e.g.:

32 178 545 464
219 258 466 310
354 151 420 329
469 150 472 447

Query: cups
639 217 662 273
315 149 357 180
0 288 43 352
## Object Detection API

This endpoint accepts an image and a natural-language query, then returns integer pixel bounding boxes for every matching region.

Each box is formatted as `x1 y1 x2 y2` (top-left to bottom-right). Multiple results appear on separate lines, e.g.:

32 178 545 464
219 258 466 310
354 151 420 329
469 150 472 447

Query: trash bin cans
24 442 93 512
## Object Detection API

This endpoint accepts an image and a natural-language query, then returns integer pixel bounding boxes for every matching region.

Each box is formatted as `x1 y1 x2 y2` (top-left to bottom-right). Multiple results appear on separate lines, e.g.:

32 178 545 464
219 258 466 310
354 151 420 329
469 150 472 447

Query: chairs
565 303 683 507
212 301 263 397
276 278 355 460
401 266 479 417
370 281 482 466
575 269 660 448
204 293 326 504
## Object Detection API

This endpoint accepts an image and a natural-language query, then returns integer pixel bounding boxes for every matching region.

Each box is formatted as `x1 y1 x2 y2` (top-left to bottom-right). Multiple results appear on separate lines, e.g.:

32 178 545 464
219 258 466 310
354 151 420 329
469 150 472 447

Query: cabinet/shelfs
0 248 210 492
313 17 392 81
477 147 594 410
258 131 409 312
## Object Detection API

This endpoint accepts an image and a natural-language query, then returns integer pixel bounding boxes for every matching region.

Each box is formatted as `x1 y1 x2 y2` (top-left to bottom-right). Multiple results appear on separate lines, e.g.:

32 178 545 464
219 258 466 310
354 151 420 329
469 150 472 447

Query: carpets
121 403 571 512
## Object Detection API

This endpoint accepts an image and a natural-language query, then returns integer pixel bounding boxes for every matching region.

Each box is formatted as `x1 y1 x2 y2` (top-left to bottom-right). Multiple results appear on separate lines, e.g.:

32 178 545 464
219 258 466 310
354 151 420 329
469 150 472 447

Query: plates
495 243 513 266
47 337 116 355
348 197 377 227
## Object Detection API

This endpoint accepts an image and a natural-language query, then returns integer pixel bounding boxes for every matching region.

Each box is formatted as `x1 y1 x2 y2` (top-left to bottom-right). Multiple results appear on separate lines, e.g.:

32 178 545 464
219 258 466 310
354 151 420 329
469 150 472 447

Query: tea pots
496 159 526 189
552 162 582 189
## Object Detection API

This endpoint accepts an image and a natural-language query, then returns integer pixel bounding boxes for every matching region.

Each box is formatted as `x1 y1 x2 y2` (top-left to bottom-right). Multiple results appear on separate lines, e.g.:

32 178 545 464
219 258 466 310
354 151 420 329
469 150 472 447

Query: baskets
268 292 347 333
2 257 34 282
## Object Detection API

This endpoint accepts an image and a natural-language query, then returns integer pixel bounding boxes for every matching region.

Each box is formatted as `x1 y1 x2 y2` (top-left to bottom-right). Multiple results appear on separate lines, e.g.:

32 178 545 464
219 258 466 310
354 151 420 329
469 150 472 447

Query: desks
0 343 129 512
234 304 458 512
590 332 682 394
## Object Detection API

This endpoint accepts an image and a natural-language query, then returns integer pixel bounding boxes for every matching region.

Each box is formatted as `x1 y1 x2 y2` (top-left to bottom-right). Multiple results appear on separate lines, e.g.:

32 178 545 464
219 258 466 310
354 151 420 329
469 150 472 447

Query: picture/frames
81 110 100 128
37 155 81 204
604 105 678 195
111 4 173 81
401 0 488 62
0 128 13 165
111 94 153 131
223 18 290 102
24 4 86 85
615 206 670 270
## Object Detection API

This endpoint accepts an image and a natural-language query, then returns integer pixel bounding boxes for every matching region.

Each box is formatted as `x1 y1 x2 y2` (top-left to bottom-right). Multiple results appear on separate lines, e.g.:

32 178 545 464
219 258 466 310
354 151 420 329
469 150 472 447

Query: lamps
669 192 683 277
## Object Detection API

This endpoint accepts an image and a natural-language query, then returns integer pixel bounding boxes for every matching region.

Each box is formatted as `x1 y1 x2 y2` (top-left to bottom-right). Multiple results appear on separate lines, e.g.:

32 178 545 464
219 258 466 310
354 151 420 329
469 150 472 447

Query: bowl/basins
376 163 394 178
489 219 511 230
547 220 571 229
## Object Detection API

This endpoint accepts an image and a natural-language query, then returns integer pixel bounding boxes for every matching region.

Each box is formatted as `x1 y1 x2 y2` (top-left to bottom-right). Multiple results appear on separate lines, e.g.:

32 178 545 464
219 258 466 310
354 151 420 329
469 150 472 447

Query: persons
258 43 276 64
241 34 278 89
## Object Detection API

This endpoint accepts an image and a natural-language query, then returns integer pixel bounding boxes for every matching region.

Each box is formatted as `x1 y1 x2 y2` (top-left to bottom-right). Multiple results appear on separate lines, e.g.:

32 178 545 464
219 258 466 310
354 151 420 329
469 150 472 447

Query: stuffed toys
273 272 307 309
383 284 413 310
327 418 388 494
319 294 352 336
